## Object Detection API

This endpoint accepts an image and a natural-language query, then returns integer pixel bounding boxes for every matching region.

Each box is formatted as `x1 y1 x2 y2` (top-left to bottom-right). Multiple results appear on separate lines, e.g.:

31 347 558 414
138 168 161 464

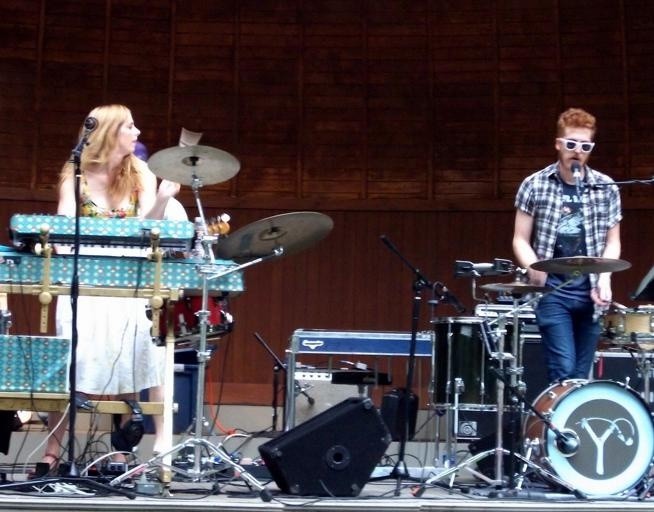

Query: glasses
556 138 595 153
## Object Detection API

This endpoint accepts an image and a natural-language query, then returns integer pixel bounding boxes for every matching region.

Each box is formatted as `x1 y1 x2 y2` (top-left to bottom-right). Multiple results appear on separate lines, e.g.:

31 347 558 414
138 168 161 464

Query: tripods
0 139 139 500
93 187 276 503
368 278 469 495
411 314 588 501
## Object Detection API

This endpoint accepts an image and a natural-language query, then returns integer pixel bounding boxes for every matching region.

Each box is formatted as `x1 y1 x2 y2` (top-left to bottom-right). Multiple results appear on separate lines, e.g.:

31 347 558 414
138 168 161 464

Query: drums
430 313 523 415
604 305 654 353
144 287 235 346
592 346 654 409
522 376 654 501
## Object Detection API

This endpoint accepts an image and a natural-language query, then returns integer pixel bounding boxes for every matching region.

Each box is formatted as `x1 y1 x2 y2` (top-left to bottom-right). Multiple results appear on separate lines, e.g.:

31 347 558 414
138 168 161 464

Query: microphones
438 281 467 313
569 162 585 196
84 116 99 129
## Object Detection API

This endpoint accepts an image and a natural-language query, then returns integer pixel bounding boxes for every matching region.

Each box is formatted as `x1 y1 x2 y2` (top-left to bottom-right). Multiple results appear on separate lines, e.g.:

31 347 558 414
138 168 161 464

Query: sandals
28 453 59 479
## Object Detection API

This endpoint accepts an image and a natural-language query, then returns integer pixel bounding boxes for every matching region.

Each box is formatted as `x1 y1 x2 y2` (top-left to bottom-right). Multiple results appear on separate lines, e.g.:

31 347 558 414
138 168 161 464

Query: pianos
0 245 245 300
11 212 197 250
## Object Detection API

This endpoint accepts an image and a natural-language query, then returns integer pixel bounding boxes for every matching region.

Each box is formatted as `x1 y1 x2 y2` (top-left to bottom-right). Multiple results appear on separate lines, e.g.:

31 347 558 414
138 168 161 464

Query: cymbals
148 144 241 187
530 255 632 276
481 281 551 297
217 211 334 259
632 264 654 298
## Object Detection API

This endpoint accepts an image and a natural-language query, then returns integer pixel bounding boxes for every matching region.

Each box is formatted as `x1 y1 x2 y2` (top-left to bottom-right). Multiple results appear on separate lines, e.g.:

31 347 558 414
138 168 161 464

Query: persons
111 140 205 463
27 103 181 486
511 107 623 386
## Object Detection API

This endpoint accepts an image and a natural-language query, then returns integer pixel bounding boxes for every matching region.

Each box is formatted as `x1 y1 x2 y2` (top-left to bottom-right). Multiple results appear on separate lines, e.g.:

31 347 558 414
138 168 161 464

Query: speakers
257 395 393 497
